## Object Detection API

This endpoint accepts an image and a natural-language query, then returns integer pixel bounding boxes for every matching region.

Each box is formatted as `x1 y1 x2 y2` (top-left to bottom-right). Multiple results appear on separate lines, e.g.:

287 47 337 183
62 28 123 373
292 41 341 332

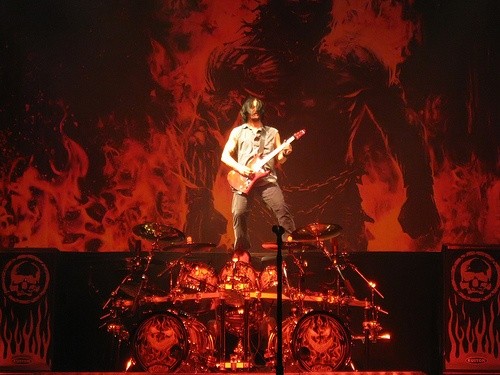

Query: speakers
0 248 63 372
439 243 500 375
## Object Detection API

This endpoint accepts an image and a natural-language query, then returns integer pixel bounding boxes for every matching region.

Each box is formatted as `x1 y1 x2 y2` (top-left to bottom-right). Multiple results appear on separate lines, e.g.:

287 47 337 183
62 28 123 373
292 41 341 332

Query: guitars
226 126 311 195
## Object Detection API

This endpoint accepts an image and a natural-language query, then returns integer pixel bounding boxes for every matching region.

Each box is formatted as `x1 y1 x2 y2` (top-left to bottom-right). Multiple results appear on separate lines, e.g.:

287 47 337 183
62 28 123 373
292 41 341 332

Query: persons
222 97 298 252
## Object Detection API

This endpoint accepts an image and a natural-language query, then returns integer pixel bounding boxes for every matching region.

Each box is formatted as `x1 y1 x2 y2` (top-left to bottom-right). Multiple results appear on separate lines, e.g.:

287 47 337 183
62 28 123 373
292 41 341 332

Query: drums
259 264 288 306
220 260 259 307
176 261 218 313
267 312 350 372
134 311 214 373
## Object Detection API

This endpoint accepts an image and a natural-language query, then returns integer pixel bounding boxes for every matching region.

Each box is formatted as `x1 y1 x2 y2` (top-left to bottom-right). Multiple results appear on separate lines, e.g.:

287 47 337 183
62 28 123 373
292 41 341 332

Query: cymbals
262 241 315 250
287 222 343 242
131 222 187 242
163 243 216 253
